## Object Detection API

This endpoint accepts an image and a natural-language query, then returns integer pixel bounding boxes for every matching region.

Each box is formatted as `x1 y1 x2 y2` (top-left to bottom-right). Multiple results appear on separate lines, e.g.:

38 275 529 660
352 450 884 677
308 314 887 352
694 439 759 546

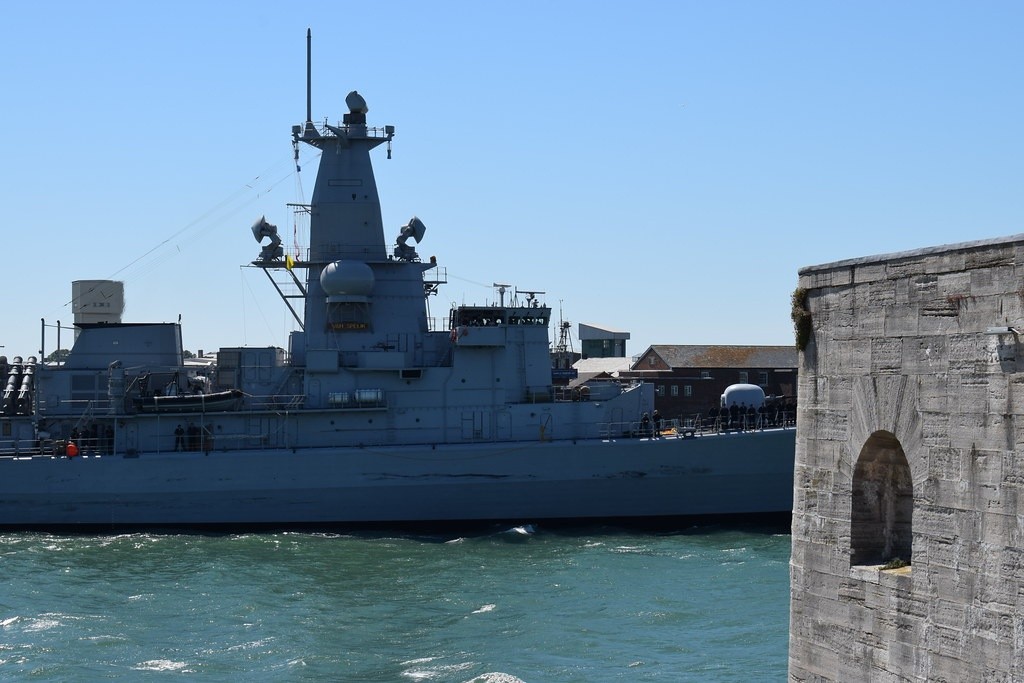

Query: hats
644 412 648 416
654 410 659 414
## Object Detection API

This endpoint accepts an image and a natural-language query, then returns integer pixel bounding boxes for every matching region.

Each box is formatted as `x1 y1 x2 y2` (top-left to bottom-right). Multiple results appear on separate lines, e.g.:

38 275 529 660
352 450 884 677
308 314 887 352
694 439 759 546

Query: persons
186 422 202 452
106 425 114 455
641 412 650 437
174 424 188 452
469 318 497 328
711 398 797 431
652 410 664 437
70 426 91 456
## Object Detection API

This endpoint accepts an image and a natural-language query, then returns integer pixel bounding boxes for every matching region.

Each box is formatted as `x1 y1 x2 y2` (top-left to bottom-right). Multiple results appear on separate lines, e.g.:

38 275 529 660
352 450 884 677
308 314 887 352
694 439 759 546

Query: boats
0 26 802 529
